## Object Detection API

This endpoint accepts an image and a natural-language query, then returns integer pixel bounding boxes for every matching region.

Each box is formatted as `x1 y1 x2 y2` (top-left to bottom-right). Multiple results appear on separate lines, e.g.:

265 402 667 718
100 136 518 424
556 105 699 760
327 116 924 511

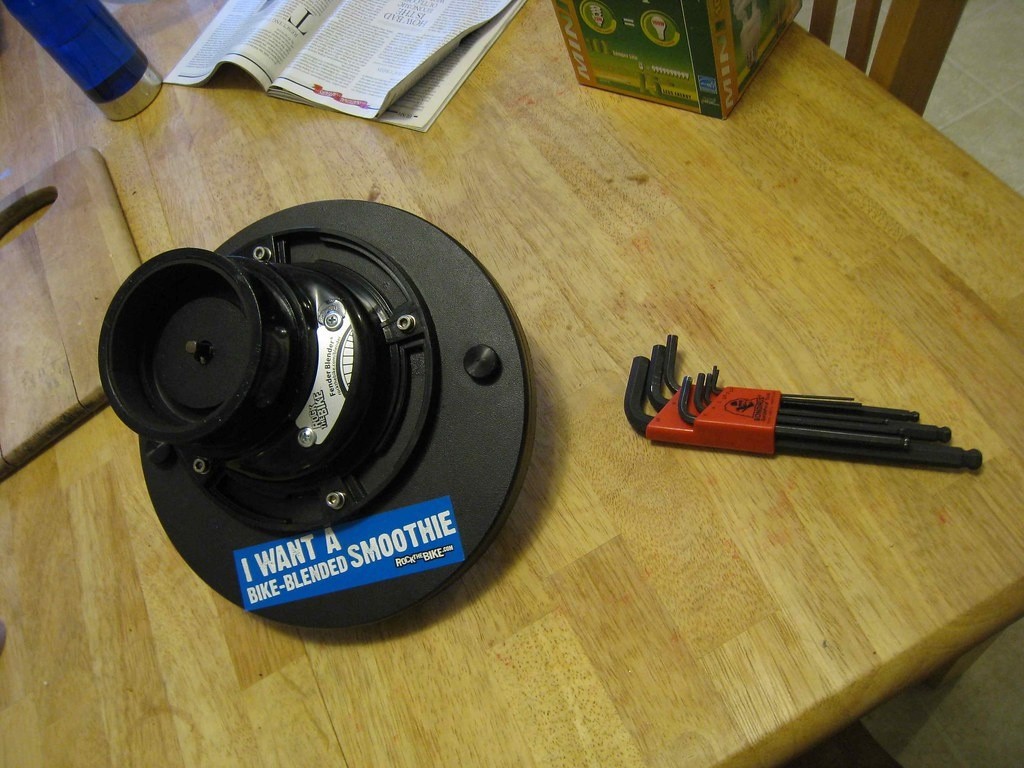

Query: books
161 0 527 132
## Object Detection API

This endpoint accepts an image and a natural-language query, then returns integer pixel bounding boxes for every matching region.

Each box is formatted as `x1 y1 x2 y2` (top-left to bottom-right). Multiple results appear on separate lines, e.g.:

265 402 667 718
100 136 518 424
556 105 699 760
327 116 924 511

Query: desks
1 2 1024 768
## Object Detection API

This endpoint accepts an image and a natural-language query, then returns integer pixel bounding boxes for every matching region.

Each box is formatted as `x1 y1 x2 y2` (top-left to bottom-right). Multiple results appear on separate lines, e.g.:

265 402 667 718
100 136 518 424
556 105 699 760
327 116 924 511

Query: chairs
808 2 966 116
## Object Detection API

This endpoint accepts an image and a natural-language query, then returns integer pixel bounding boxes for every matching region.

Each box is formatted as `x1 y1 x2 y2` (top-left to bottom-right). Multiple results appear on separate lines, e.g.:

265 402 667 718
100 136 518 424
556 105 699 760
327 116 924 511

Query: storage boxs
554 0 804 121
